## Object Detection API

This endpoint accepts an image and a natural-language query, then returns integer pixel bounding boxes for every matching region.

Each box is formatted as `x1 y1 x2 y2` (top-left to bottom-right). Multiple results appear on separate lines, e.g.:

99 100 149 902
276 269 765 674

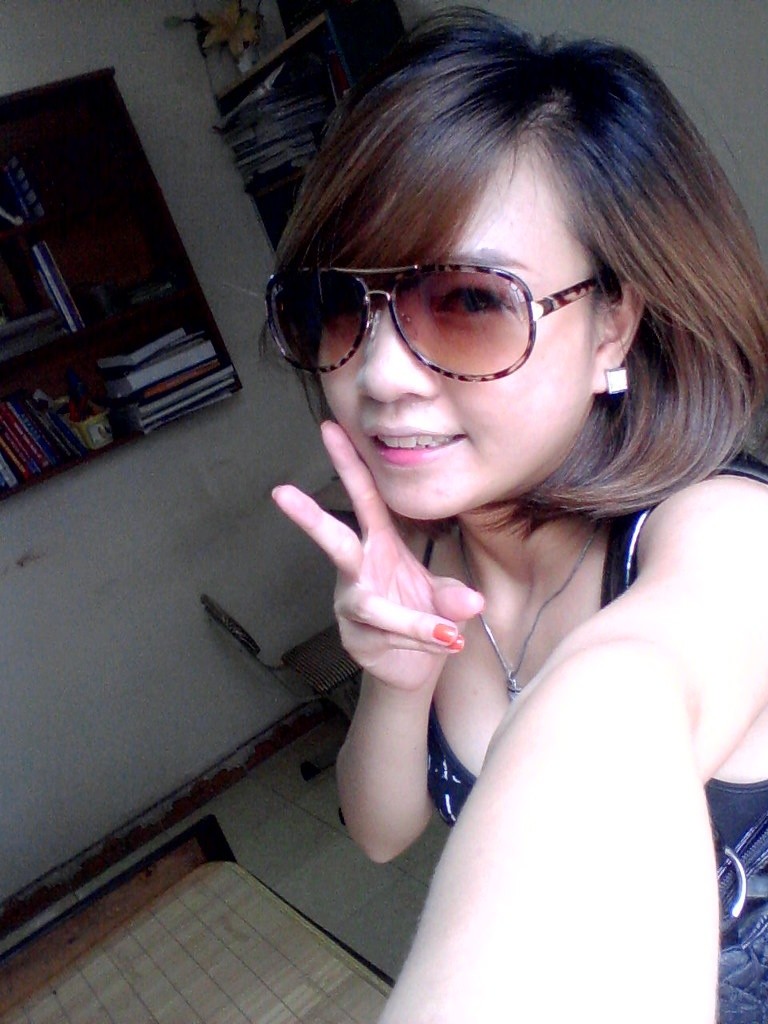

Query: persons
272 23 766 1024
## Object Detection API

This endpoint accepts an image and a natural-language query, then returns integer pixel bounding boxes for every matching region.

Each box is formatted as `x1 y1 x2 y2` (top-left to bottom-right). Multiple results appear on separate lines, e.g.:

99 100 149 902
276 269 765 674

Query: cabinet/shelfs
1 66 243 505
188 0 407 262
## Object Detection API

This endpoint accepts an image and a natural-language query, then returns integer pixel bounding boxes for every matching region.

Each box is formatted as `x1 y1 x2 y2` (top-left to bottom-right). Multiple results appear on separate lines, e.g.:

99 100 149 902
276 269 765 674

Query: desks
307 474 449 573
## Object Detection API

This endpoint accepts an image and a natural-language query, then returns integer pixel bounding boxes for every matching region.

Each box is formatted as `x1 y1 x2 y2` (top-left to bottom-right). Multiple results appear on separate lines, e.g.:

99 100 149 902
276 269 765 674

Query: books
211 57 330 192
0 322 236 492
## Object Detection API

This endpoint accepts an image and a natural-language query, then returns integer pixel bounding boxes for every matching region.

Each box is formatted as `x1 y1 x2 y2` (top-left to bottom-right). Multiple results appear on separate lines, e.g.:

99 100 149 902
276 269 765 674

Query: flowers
162 1 264 57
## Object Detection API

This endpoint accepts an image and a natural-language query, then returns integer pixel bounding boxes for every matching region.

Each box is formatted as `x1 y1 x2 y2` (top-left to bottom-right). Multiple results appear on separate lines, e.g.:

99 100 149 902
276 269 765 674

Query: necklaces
455 522 602 706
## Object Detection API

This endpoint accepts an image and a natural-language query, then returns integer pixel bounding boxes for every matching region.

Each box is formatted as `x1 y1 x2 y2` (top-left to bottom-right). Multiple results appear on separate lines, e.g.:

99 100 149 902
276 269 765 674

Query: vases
236 47 252 71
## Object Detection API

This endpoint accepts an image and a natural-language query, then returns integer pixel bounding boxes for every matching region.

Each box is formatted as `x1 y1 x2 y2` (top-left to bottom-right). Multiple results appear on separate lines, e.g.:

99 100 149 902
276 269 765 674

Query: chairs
197 592 363 827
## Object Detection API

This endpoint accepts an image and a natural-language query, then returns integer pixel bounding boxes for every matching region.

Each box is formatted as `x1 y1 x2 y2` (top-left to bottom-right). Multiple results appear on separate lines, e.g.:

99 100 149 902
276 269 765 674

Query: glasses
263 255 606 386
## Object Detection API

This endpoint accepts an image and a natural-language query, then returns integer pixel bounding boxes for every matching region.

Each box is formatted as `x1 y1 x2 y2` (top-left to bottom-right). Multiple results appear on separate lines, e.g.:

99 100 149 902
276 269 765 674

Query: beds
0 813 396 1023
